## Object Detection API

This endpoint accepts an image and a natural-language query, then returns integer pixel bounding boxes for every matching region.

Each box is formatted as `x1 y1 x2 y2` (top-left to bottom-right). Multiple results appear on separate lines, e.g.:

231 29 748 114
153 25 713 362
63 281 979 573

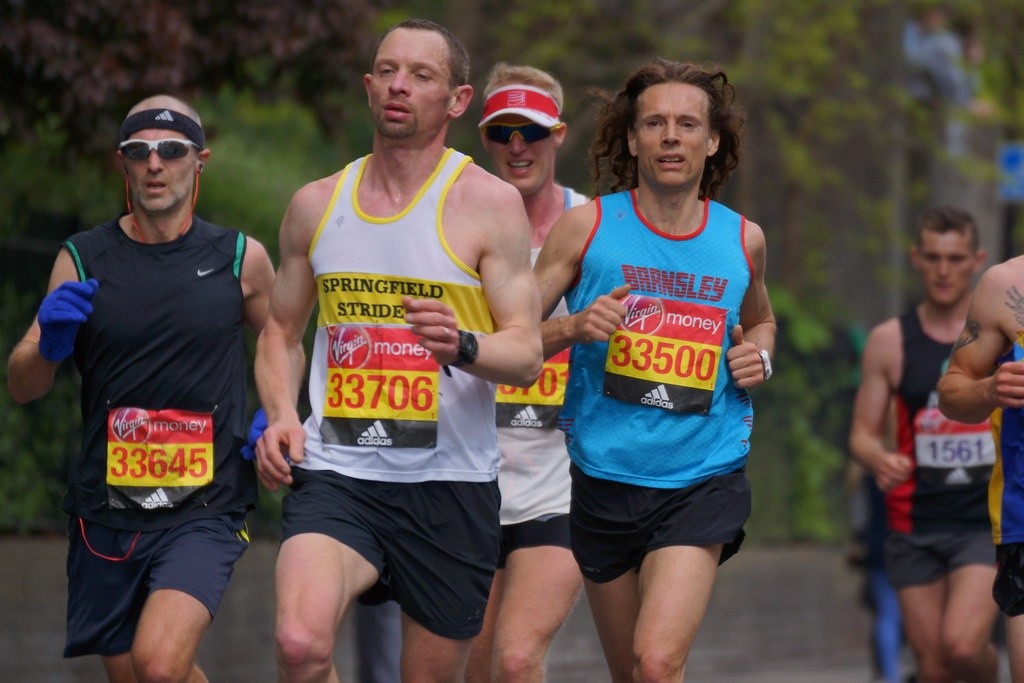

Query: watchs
443 330 479 377
758 348 772 380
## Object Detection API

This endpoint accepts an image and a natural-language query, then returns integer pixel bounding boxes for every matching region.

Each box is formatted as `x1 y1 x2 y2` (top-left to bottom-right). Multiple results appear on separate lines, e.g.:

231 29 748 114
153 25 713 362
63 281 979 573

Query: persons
533 58 775 683
254 21 544 683
8 97 304 683
464 63 593 683
845 208 1003 683
938 256 1024 683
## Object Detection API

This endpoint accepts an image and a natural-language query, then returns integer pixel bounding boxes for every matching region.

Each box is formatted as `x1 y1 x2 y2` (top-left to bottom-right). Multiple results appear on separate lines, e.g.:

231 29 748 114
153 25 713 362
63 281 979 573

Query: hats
478 85 559 127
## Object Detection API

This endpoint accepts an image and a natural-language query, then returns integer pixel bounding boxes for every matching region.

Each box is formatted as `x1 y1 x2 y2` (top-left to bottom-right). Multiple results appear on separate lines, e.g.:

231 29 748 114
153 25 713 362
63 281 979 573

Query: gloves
37 278 100 362
240 408 290 464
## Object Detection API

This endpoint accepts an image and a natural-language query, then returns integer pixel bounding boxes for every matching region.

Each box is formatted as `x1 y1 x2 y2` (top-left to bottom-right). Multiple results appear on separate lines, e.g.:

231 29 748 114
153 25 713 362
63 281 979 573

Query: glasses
479 121 560 145
119 138 202 160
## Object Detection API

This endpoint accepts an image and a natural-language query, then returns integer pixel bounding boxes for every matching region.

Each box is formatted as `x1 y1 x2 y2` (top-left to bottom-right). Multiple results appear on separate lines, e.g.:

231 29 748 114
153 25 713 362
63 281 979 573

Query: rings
445 327 450 338
